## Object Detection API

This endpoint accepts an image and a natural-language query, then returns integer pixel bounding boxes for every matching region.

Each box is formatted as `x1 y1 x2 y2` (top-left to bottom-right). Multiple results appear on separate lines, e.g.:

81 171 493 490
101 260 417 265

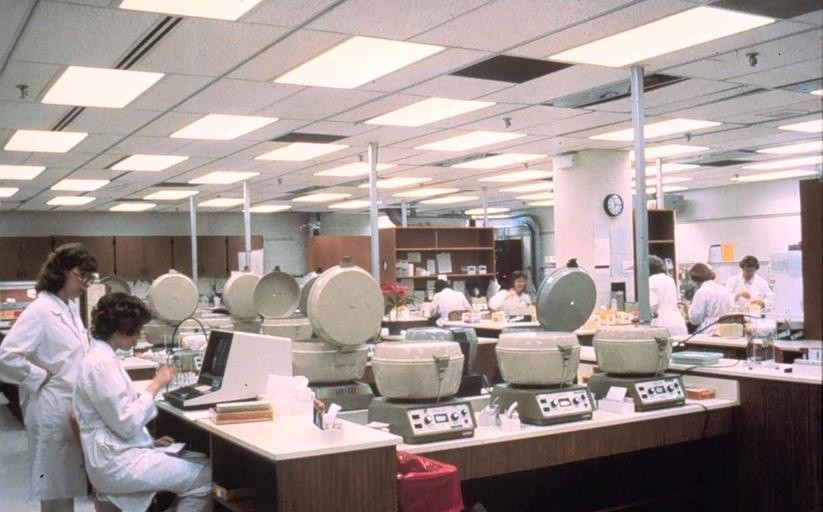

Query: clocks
604 193 623 216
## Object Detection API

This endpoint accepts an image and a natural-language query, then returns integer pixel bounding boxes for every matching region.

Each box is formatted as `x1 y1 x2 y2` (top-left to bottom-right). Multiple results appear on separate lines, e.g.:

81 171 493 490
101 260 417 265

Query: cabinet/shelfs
379 226 496 304
173 233 227 281
153 397 404 512
632 208 680 300
115 236 172 283
0 236 52 283
53 235 110 287
228 235 263 279
307 235 371 276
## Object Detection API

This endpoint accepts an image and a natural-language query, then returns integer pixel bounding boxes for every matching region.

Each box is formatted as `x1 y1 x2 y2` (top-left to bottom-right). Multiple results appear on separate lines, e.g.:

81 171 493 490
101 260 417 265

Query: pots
371 339 465 399
593 324 671 375
510 259 594 328
144 269 200 343
300 263 323 319
495 330 579 384
293 254 386 384
222 274 259 330
254 265 310 339
103 274 131 300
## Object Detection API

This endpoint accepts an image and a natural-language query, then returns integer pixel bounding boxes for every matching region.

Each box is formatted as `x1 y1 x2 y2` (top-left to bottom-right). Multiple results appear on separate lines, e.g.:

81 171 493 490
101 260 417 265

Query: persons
72 294 212 512
725 255 775 315
488 271 531 319
0 242 98 512
428 280 472 327
648 255 688 336
683 263 731 335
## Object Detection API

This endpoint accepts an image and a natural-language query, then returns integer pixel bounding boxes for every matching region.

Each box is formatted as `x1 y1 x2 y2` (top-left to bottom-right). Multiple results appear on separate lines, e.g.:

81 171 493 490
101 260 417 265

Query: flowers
380 282 410 320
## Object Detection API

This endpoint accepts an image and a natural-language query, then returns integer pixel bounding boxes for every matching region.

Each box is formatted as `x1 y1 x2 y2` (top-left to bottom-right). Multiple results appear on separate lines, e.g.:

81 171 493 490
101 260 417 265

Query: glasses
69 269 95 287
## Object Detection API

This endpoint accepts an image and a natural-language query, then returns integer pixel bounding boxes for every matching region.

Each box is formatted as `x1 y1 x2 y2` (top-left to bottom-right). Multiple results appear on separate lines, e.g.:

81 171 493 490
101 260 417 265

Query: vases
389 321 403 335
